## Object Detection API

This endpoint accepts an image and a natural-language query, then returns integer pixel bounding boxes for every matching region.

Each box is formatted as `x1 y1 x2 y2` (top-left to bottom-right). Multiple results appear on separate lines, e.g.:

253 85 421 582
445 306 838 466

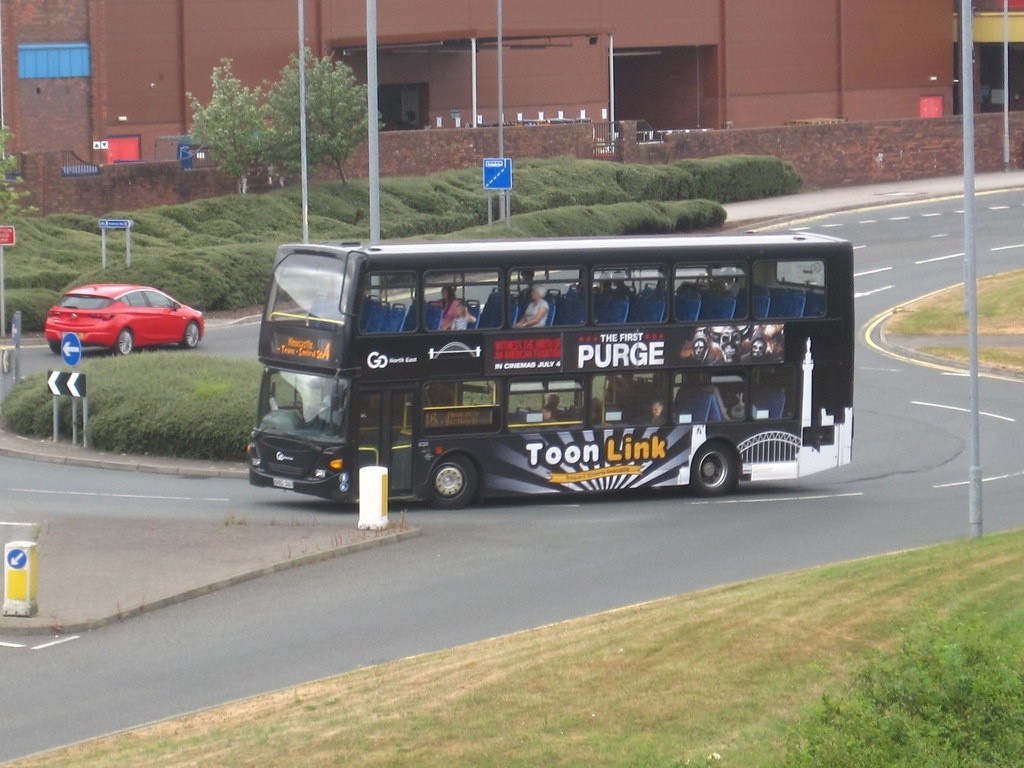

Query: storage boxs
304 277 823 334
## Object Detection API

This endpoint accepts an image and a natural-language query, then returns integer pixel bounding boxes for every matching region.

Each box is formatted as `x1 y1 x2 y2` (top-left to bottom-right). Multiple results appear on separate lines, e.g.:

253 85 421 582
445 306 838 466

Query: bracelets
522 323 526 327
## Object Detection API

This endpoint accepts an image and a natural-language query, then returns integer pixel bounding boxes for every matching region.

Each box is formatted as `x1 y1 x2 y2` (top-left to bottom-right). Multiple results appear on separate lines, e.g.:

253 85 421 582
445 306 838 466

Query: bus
248 229 855 505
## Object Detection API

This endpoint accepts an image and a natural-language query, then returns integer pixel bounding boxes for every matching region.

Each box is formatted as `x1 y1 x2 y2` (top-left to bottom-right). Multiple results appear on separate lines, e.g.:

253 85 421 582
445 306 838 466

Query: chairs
622 378 785 424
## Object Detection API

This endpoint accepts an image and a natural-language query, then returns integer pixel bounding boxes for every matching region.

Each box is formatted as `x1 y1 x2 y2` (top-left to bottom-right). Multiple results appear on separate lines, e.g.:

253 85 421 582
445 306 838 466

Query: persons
648 396 668 426
515 284 549 328
319 396 343 435
612 271 632 298
544 394 562 422
438 284 477 331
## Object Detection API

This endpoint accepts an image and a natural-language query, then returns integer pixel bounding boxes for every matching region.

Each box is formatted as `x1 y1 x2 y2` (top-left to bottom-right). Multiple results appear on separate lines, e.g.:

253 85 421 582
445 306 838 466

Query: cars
43 284 204 357
